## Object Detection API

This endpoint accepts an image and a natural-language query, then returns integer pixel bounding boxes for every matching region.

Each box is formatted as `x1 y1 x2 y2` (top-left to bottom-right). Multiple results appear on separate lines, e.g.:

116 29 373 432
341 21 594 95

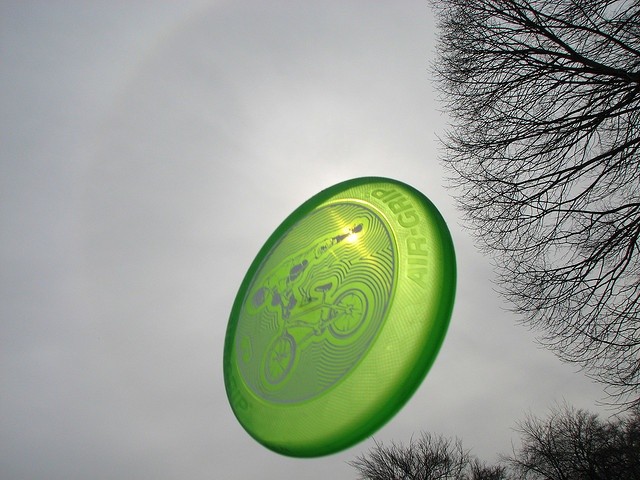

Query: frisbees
223 176 456 458
